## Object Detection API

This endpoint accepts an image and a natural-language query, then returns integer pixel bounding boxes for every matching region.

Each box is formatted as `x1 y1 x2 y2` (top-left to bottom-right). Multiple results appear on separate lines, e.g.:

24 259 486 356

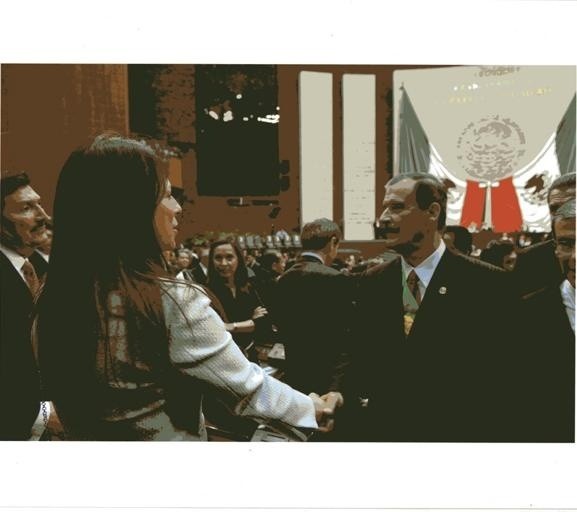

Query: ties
21 261 41 299
404 269 420 313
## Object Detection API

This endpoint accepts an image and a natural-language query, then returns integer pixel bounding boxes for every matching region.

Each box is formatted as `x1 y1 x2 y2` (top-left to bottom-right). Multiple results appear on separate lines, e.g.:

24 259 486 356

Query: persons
1 131 577 441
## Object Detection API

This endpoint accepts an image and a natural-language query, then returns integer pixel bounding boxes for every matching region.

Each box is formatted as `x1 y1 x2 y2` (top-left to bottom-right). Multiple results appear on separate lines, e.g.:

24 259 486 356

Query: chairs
238 235 303 257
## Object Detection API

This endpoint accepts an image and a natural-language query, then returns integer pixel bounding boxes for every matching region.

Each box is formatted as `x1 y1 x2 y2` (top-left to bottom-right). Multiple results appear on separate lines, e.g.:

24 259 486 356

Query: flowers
192 231 215 249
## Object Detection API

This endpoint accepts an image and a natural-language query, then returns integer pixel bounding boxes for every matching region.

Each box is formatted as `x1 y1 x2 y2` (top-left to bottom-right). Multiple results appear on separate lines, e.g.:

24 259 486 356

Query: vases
193 247 210 256
